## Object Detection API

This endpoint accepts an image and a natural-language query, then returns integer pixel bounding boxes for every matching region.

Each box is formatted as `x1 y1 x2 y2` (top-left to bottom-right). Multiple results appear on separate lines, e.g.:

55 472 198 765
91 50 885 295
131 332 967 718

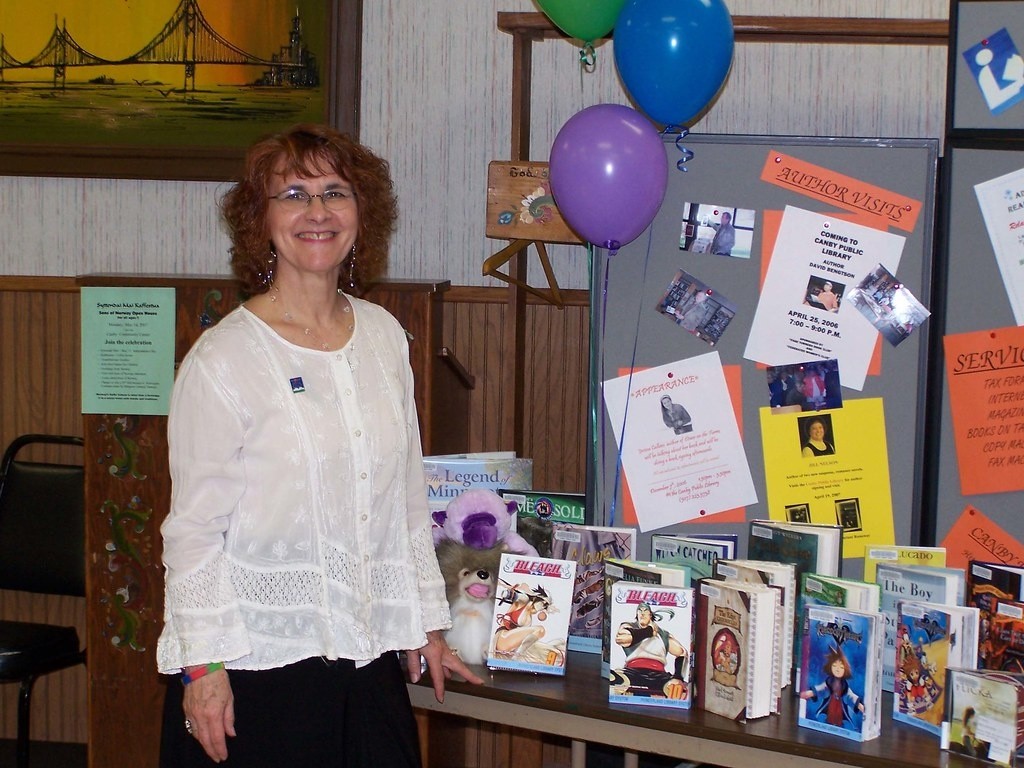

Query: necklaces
269 281 354 351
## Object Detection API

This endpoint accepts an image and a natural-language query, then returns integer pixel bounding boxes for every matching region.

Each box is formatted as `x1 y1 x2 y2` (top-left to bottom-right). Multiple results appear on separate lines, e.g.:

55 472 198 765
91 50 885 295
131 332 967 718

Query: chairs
0 433 85 768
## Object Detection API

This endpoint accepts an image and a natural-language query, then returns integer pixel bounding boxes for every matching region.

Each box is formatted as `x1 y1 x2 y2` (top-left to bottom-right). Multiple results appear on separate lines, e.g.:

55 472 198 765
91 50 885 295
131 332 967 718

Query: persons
157 123 485 768
660 212 915 459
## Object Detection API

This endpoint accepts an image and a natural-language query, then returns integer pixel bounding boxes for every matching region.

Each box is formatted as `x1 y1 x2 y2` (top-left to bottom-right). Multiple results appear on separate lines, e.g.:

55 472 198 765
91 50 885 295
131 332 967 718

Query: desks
398 645 1022 768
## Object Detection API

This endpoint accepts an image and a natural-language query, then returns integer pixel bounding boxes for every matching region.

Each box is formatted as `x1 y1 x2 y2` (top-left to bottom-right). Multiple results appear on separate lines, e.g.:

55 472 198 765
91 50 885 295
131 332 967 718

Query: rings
450 648 459 656
184 719 198 735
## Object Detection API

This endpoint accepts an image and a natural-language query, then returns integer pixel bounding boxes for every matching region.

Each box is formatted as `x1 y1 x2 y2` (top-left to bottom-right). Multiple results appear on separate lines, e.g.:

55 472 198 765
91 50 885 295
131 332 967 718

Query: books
422 452 1024 768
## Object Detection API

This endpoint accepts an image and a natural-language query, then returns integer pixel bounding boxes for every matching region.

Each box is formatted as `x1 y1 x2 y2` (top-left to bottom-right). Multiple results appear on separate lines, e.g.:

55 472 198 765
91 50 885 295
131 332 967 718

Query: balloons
534 0 734 256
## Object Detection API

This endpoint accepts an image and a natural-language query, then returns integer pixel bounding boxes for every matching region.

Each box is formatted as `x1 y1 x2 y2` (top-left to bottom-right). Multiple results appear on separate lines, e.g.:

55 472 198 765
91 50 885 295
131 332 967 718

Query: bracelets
179 661 225 687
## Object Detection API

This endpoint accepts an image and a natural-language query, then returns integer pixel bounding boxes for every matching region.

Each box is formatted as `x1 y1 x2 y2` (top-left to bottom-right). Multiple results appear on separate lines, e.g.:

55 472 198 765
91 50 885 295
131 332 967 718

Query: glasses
268 189 357 212
